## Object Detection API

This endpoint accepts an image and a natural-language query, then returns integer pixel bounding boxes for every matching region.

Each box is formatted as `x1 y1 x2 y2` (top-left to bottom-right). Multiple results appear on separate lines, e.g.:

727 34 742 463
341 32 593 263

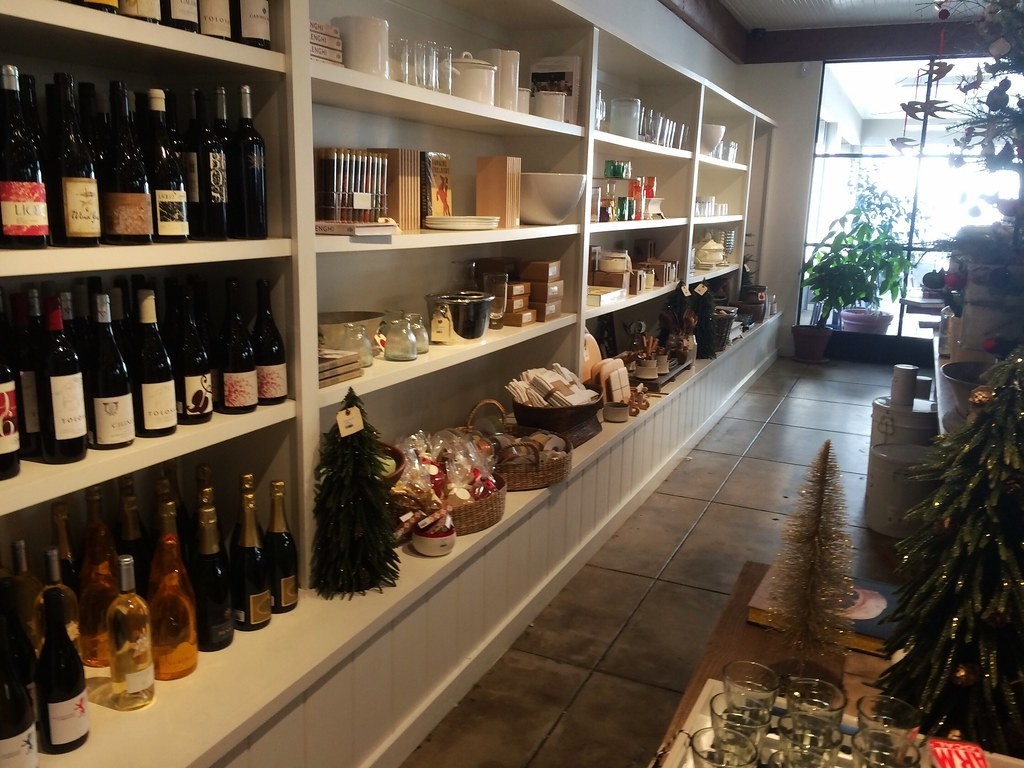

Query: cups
340 259 509 367
331 14 566 123
892 363 918 405
624 286 777 379
690 227 736 274
591 91 739 224
915 375 932 400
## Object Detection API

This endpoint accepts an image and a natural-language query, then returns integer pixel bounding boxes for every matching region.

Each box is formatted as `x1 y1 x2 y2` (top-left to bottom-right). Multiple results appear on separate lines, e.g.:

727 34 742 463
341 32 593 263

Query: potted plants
791 178 930 364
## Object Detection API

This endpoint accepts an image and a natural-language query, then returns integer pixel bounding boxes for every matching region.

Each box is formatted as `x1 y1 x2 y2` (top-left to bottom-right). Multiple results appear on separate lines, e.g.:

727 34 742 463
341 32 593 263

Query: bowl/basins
729 301 765 323
700 124 726 155
377 440 406 490
520 171 588 226
940 360 1000 419
316 311 386 357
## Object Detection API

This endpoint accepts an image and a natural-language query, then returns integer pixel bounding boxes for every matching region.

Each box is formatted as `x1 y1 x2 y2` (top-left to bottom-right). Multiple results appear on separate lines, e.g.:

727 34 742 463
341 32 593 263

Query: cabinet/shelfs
1 0 778 767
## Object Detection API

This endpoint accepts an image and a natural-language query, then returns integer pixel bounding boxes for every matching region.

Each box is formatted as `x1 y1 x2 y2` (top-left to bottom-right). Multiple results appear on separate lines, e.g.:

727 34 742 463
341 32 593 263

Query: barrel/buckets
871 395 940 445
865 444 937 538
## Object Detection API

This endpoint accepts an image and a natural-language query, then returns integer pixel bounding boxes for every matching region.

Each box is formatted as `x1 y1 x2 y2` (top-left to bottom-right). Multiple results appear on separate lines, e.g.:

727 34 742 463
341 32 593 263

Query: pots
693 232 724 263
598 249 628 273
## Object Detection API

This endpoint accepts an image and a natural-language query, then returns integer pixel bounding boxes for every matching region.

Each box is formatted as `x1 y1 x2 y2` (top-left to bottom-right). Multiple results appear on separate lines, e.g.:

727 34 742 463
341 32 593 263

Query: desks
933 328 977 439
644 561 894 768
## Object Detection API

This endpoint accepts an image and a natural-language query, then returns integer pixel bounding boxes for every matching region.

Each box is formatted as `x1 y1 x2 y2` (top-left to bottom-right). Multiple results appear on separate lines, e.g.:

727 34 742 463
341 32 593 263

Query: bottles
0 468 299 759
0 259 287 480
0 61 269 251
60 0 271 51
938 290 958 357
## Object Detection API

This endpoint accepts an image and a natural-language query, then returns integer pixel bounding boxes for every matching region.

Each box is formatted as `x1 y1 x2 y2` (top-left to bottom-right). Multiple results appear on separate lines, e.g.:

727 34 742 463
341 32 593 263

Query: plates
425 214 500 231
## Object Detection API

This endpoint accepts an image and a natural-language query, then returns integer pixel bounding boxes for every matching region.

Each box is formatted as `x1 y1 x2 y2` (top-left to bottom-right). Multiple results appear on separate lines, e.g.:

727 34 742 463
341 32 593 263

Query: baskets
466 399 573 490
448 472 507 537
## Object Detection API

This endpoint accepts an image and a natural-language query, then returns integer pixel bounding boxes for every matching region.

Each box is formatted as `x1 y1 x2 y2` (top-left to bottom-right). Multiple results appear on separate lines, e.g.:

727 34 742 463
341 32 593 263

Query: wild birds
899 100 955 121
890 137 918 153
919 62 955 84
